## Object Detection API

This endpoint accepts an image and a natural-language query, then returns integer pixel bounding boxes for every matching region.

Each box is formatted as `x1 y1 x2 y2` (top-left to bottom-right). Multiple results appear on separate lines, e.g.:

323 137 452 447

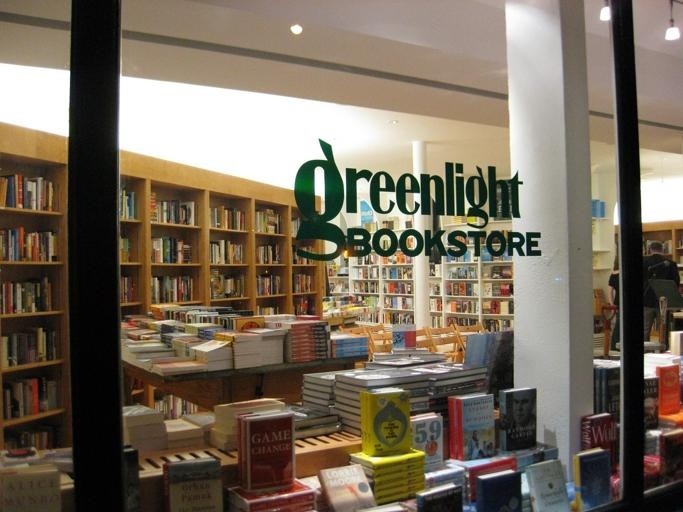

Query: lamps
664 0 681 41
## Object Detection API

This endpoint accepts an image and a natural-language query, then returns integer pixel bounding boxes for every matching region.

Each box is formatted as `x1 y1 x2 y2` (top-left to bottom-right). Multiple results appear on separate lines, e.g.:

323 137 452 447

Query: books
1 169 59 450
1 446 75 512
121 183 682 510
